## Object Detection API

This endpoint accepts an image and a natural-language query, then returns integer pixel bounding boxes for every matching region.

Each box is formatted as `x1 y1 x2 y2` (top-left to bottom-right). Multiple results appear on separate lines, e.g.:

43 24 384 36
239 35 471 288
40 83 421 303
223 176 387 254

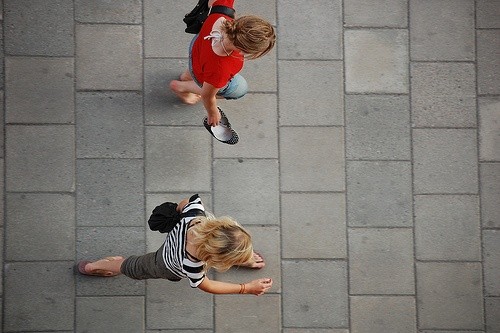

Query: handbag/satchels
184 0 235 34
148 202 206 234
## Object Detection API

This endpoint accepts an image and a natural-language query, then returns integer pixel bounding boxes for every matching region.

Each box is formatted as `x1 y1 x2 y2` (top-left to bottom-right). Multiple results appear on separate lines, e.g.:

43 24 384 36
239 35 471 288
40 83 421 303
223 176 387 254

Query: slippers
79 257 122 276
233 252 264 270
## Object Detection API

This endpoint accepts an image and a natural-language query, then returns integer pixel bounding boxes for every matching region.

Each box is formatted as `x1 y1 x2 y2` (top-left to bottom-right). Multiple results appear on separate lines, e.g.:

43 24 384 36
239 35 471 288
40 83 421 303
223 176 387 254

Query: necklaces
220 30 234 56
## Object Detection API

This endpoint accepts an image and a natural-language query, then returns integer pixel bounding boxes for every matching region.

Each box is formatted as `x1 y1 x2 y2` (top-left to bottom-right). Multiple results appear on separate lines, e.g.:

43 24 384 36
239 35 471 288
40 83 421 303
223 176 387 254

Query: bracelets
242 283 246 294
239 283 243 294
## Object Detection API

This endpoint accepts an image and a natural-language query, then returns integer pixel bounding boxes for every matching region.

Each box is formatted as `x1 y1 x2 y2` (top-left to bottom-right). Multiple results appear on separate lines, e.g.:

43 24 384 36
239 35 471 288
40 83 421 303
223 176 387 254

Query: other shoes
203 118 239 146
215 107 233 129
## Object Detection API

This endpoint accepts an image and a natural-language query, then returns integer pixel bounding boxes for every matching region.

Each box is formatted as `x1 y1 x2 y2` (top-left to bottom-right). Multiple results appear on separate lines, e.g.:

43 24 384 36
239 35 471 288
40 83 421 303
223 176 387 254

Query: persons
169 0 277 128
76 193 274 299
